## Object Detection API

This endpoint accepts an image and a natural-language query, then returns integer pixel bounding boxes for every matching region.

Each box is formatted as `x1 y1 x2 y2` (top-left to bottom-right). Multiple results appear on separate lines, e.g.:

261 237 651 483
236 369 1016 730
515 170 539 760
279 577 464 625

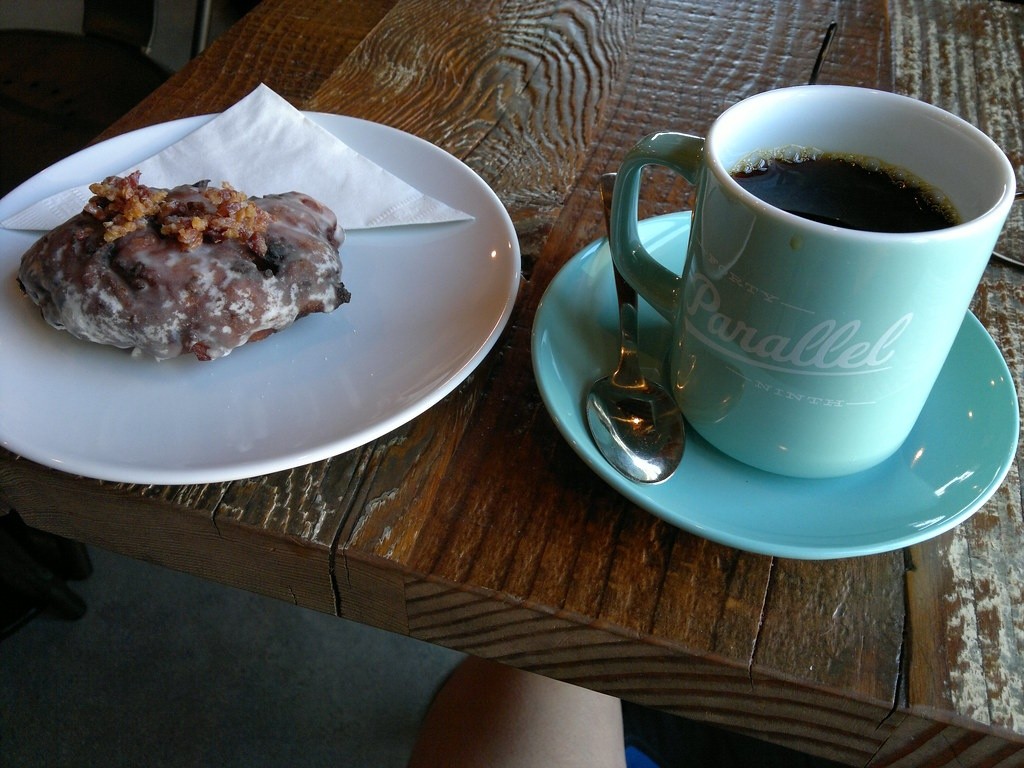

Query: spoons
587 172 687 486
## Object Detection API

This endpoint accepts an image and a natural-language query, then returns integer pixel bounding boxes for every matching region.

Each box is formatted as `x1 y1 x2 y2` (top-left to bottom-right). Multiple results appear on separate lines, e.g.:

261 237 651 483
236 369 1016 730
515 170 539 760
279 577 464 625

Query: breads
20 172 351 359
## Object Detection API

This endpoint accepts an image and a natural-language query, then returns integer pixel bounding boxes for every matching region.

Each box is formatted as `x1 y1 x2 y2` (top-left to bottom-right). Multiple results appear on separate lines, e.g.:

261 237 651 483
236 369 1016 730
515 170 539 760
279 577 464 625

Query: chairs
0 0 214 198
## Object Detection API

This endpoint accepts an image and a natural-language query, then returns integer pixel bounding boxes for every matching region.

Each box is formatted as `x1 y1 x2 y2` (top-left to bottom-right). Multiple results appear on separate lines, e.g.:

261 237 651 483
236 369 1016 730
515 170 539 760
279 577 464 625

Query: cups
610 84 1017 478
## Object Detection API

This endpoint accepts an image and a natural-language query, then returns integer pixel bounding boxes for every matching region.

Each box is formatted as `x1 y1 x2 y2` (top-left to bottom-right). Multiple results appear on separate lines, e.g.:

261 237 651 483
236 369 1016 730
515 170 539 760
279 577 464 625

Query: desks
0 0 1024 768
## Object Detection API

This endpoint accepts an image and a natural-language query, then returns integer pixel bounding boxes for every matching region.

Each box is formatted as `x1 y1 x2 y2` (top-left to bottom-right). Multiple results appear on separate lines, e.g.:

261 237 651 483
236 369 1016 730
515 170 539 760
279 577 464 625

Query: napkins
1 81 477 231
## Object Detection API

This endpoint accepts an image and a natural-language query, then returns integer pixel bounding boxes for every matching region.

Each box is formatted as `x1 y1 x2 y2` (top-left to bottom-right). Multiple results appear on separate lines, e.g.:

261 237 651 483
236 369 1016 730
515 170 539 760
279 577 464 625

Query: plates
0 110 521 484
532 210 1020 559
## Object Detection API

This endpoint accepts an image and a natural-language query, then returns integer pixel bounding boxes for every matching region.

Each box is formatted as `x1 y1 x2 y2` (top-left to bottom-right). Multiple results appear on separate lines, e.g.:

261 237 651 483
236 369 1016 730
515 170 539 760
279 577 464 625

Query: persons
403 653 661 768
